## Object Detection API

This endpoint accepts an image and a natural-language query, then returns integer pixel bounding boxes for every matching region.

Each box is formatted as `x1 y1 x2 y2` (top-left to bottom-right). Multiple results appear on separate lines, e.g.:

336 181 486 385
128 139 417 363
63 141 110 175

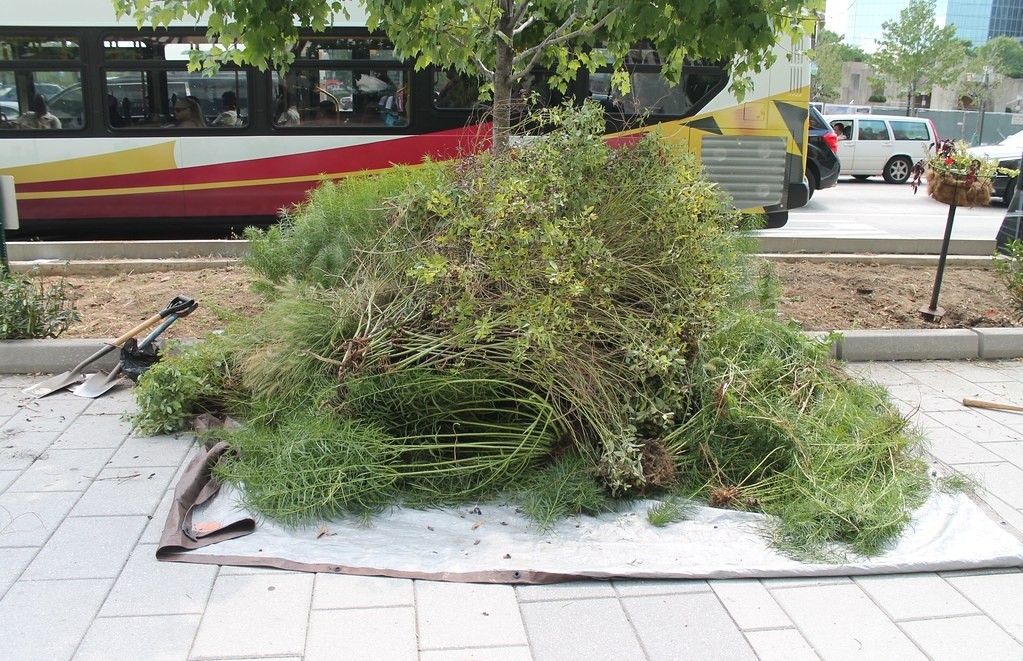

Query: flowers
933 144 1020 180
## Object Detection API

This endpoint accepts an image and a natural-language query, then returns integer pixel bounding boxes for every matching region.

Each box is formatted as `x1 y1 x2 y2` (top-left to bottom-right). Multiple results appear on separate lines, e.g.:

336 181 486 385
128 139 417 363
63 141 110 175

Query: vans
823 114 942 184
809 102 872 114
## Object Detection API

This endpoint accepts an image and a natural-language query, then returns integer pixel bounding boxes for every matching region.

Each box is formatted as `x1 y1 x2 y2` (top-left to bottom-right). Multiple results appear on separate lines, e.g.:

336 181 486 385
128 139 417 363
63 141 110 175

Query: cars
953 130 1023 207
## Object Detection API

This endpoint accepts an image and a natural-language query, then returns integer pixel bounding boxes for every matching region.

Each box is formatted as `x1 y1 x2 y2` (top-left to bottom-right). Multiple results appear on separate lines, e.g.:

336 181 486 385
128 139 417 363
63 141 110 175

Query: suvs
805 104 841 201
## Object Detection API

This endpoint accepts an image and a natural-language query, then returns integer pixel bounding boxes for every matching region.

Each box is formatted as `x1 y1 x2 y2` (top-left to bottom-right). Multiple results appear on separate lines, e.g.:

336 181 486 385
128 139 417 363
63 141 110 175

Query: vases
926 170 991 206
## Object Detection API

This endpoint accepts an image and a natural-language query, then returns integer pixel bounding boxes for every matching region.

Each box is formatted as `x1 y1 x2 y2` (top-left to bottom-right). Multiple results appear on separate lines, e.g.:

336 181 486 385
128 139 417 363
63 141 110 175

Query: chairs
110 72 479 130
843 125 851 140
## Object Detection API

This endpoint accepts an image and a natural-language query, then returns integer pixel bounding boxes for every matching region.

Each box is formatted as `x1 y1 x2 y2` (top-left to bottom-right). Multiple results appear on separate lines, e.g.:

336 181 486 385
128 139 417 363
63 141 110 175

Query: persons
359 69 397 124
315 100 336 125
834 123 846 140
435 71 469 108
19 93 62 129
221 90 240 116
174 96 208 128
275 76 296 120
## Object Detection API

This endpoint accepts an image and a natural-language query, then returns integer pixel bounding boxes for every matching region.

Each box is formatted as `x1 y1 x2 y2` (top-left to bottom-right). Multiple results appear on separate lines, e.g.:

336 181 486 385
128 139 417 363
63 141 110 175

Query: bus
1 1 813 231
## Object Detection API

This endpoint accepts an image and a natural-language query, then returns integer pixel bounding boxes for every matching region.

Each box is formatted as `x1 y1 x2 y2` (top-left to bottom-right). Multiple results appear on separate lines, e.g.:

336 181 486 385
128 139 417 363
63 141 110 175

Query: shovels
20 294 199 399
